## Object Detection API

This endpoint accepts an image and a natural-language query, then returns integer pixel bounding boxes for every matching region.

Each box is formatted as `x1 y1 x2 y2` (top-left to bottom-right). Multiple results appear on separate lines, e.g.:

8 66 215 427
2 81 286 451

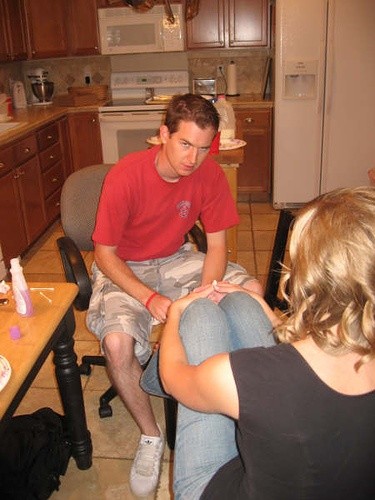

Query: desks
0 279 94 471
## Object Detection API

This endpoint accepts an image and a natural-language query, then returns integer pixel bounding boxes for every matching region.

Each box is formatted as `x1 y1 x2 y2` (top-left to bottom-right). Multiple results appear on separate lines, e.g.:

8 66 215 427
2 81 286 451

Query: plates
0 354 12 392
218 138 247 150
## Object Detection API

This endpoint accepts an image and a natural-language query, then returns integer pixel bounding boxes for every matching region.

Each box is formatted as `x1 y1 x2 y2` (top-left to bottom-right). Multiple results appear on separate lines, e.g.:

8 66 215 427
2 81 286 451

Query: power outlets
217 65 224 77
84 74 92 86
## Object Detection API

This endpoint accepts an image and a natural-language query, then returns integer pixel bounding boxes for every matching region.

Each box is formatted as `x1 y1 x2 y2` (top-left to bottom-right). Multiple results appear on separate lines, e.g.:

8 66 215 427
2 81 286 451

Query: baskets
68 85 108 100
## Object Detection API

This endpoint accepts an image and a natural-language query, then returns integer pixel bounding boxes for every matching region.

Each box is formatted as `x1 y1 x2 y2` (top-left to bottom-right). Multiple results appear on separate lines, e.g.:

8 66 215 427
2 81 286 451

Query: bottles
212 94 235 144
9 258 34 318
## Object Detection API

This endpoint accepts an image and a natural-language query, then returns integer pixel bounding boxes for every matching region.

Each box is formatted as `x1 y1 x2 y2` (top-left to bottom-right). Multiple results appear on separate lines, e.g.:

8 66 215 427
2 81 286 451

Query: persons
86 92 261 498
141 187 375 500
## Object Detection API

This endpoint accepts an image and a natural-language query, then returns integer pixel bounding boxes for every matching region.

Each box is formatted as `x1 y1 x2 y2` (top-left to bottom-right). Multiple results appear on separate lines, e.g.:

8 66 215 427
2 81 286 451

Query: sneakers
129 423 165 498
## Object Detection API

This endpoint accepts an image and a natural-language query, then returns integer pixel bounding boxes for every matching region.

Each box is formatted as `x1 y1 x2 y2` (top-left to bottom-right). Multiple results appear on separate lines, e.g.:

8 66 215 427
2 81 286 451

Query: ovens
99 111 167 164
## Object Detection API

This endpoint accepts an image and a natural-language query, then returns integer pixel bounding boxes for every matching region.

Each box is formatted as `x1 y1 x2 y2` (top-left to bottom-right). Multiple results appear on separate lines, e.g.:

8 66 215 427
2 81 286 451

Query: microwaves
98 3 184 55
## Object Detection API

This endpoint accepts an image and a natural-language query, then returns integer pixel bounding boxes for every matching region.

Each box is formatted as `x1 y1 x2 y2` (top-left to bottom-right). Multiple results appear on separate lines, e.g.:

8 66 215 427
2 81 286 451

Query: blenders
25 67 54 105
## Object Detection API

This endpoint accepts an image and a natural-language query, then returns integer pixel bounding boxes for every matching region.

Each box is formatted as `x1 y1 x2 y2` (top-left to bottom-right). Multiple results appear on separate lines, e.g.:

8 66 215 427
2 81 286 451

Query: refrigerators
271 0 375 210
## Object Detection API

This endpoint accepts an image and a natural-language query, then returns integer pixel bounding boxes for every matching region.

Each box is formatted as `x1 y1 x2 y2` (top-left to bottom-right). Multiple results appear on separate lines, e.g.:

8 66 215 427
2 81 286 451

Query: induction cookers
98 71 189 112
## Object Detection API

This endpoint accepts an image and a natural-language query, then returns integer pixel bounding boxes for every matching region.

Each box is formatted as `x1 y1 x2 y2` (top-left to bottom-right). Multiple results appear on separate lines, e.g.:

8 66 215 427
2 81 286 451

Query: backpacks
0 407 72 500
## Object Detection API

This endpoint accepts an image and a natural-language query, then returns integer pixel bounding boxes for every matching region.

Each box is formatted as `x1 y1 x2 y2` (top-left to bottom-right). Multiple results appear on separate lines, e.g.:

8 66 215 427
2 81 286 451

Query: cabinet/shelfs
229 108 273 196
0 0 99 62
185 0 271 49
0 111 100 272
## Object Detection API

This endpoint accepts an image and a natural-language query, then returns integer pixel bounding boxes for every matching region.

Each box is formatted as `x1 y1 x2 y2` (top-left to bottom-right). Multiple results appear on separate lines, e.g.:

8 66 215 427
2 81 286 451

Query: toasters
193 78 217 100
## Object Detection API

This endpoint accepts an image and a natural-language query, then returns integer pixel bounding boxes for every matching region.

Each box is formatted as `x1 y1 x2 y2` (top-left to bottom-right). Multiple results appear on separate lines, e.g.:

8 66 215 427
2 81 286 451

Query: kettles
13 81 27 108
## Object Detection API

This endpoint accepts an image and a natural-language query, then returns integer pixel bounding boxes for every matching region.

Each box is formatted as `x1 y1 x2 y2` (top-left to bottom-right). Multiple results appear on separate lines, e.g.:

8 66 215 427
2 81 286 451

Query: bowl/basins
31 83 54 101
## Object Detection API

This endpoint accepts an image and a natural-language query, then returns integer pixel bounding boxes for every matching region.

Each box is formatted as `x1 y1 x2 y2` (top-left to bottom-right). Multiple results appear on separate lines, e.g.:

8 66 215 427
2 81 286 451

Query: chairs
57 163 208 449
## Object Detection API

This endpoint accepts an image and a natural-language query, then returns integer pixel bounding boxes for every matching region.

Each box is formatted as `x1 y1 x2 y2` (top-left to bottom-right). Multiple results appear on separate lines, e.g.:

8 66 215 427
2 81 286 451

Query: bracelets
145 292 157 308
